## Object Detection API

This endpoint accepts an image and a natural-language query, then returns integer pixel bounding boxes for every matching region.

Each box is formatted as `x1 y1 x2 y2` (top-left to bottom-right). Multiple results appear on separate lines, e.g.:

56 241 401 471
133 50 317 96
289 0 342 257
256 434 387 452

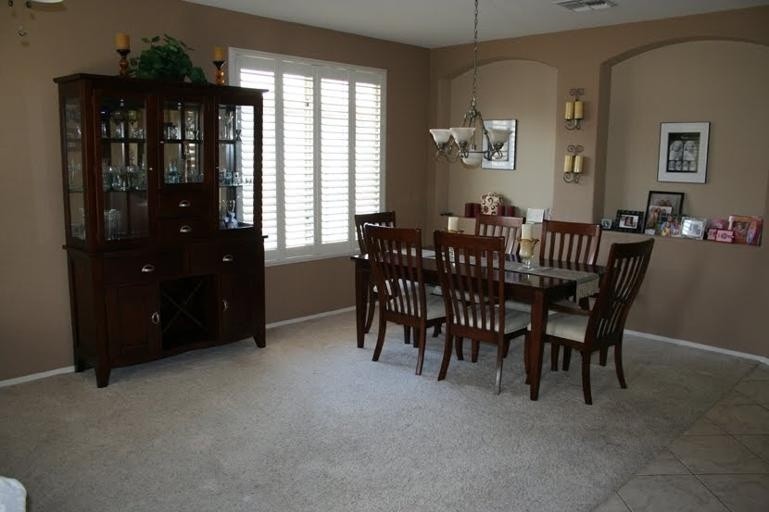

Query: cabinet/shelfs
101 251 165 284
157 187 215 217
102 282 166 368
219 274 266 348
188 243 264 276
210 94 262 243
158 92 214 188
90 84 156 250
157 218 215 247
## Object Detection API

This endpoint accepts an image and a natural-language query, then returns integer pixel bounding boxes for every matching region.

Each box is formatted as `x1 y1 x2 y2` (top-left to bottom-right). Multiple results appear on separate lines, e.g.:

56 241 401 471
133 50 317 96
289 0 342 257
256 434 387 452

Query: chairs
354 210 440 348
538 218 601 372
524 237 656 407
363 224 464 377
472 213 527 358
432 229 533 394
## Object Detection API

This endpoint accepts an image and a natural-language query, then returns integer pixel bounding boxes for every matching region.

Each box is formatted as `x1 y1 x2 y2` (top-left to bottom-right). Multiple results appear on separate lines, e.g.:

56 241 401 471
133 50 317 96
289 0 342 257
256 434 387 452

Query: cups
104 209 120 241
222 200 235 217
125 164 137 189
110 165 122 190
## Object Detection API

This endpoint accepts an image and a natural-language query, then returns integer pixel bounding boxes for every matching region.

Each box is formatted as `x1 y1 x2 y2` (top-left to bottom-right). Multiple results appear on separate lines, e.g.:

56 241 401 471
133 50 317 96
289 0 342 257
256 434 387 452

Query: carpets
2 284 765 511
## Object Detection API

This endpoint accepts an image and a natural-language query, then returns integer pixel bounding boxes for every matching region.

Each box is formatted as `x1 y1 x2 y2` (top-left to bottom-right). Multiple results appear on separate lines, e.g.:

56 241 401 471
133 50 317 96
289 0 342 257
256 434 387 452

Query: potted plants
130 36 206 92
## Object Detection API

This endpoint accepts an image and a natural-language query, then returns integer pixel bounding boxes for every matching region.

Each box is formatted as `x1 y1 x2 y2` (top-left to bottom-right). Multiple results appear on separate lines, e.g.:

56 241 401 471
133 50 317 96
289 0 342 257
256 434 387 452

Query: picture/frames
727 214 764 247
656 120 711 185
679 215 704 242
479 118 518 171
642 190 686 237
613 208 645 234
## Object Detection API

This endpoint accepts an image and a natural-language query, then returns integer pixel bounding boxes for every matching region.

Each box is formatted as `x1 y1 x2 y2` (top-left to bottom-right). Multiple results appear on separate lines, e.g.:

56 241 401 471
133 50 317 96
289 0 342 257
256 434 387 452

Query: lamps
562 144 586 185
426 1 511 168
562 87 585 131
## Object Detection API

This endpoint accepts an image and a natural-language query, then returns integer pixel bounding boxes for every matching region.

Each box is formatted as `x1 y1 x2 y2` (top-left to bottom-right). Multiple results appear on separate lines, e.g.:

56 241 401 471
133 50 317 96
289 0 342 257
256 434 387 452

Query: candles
112 30 134 53
211 43 228 64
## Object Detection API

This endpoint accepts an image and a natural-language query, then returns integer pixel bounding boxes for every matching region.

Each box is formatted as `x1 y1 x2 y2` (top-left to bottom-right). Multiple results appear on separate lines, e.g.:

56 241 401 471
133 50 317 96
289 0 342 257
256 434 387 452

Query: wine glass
221 110 233 141
